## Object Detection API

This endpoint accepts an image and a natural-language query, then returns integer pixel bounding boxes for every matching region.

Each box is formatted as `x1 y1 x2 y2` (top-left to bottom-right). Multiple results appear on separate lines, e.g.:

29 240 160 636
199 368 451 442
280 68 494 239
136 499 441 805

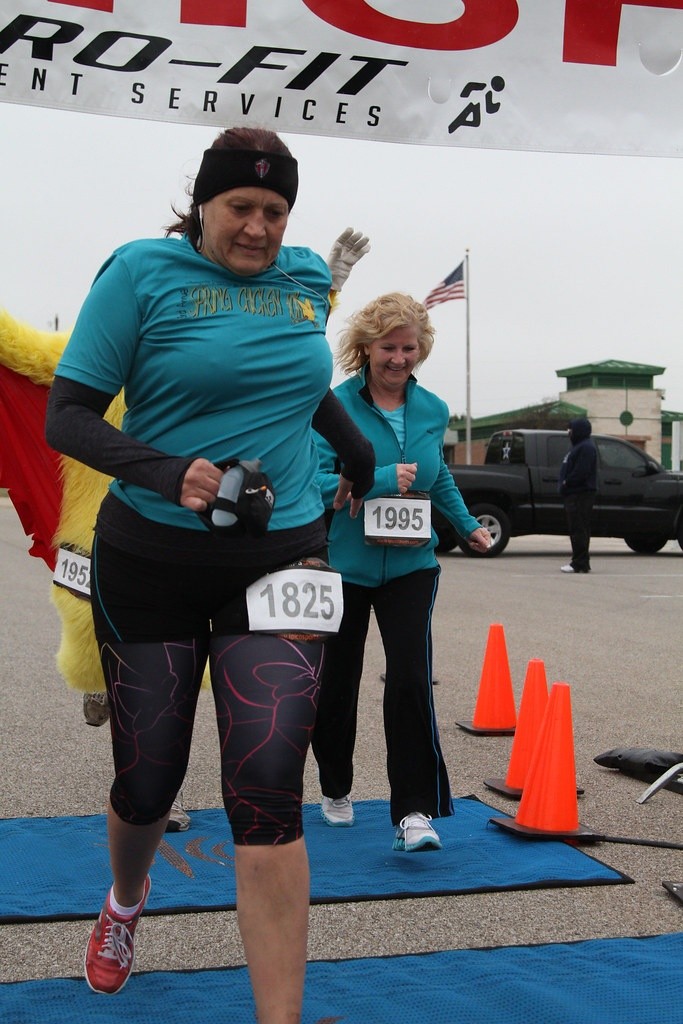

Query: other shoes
562 565 574 573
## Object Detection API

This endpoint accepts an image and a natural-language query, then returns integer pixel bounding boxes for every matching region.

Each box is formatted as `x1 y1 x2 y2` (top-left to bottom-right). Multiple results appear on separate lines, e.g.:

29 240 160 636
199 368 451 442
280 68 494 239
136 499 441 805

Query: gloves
323 228 372 292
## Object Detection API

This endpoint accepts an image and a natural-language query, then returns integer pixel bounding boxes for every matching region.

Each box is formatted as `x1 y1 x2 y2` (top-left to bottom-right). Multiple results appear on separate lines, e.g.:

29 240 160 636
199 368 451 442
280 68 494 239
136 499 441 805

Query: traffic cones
481 655 587 801
454 622 519 737
487 682 607 844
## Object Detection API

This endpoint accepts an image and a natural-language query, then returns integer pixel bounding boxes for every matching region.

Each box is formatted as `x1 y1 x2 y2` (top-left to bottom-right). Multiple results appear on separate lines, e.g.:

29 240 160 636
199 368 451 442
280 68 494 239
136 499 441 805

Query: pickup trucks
429 427 683 558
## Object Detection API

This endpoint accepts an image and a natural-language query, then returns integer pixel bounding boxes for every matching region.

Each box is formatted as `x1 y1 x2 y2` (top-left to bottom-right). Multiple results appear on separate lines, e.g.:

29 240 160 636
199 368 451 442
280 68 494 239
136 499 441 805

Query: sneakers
164 783 190 833
83 693 109 726
84 874 151 994
392 813 442 851
320 794 354 827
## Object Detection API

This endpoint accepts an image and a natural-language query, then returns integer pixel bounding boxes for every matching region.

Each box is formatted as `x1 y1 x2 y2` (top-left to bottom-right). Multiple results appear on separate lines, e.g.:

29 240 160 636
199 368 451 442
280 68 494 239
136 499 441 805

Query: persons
41 125 375 1024
558 420 599 574
3 223 372 833
309 292 490 854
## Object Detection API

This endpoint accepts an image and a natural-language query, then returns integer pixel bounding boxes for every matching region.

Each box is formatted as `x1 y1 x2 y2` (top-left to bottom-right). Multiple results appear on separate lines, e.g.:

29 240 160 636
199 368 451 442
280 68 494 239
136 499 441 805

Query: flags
420 262 466 311
